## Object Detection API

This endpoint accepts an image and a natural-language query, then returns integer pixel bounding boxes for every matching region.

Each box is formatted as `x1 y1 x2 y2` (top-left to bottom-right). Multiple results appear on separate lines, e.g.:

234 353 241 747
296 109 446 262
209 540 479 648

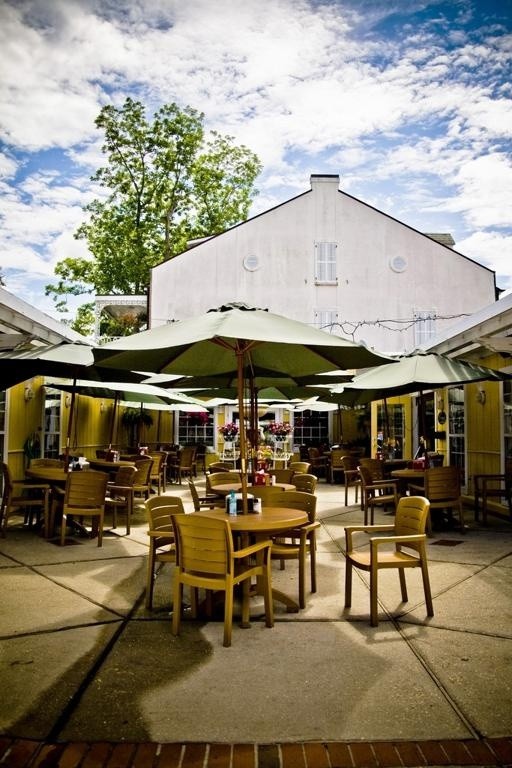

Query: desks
190 507 308 628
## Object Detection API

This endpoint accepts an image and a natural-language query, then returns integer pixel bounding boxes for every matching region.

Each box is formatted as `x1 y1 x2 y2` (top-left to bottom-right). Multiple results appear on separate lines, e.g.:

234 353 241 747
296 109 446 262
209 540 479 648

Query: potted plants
119 405 155 449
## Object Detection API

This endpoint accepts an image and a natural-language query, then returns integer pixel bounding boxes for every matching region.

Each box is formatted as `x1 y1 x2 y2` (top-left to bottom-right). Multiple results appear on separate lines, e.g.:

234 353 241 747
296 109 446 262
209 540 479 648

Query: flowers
218 422 241 443
263 420 300 442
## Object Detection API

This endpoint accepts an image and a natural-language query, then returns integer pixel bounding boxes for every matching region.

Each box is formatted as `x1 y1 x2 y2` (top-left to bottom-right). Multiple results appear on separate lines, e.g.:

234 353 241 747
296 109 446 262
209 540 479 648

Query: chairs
308 440 370 486
264 492 321 610
340 452 511 533
189 441 319 511
170 514 274 647
1 440 206 548
144 495 199 620
345 496 434 628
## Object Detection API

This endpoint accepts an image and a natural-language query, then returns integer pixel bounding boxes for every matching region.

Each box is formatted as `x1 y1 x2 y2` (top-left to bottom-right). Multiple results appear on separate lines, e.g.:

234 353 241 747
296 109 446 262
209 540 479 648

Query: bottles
252 497 262 513
229 488 237 516
